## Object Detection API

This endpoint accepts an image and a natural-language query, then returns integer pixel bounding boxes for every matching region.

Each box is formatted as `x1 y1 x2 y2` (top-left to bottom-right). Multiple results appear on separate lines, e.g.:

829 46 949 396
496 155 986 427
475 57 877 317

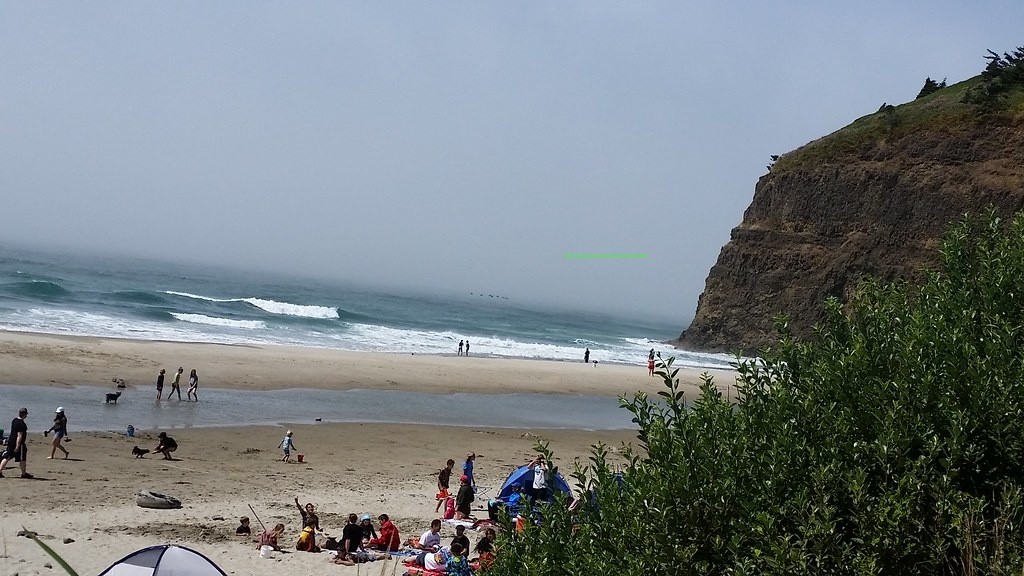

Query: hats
460 474 468 482
19 408 28 415
361 514 371 522
54 407 64 413
158 432 167 438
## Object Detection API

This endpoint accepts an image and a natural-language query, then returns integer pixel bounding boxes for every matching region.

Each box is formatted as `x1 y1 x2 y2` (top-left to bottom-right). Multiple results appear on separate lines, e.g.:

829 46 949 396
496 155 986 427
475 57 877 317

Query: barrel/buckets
65 437 68 440
298 455 304 462
127 430 134 436
593 363 596 368
260 545 274 559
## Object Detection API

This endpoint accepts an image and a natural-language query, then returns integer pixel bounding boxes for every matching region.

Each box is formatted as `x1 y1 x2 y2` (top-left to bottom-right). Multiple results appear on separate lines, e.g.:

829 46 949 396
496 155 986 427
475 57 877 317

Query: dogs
104 391 123 404
131 445 150 458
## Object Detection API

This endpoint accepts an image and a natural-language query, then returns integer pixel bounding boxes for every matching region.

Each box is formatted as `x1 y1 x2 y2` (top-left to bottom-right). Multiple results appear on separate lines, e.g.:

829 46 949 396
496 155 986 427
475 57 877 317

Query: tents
499 465 573 506
97 544 226 576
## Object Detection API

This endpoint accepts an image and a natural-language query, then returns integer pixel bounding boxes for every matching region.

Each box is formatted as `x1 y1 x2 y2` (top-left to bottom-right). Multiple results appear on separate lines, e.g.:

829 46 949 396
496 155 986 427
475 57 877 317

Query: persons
0 408 33 478
46 407 70 459
187 369 198 401
235 516 251 536
529 454 548 502
168 367 183 400
402 518 470 570
458 340 464 356
488 483 521 520
152 432 178 459
466 340 469 356
278 431 296 463
324 513 401 566
435 452 475 519
157 369 165 400
472 528 495 570
260 524 284 551
648 349 655 377
585 348 590 363
295 496 323 553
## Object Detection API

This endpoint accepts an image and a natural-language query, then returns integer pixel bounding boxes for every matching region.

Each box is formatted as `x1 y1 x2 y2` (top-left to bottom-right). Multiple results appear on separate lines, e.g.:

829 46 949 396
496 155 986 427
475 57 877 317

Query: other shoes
0 473 5 478
21 472 33 479
44 430 47 437
64 439 72 442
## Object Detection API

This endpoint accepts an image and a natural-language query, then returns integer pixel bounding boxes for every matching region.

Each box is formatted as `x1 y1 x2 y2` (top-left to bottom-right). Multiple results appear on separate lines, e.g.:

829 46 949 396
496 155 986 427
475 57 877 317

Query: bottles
44 431 48 437
